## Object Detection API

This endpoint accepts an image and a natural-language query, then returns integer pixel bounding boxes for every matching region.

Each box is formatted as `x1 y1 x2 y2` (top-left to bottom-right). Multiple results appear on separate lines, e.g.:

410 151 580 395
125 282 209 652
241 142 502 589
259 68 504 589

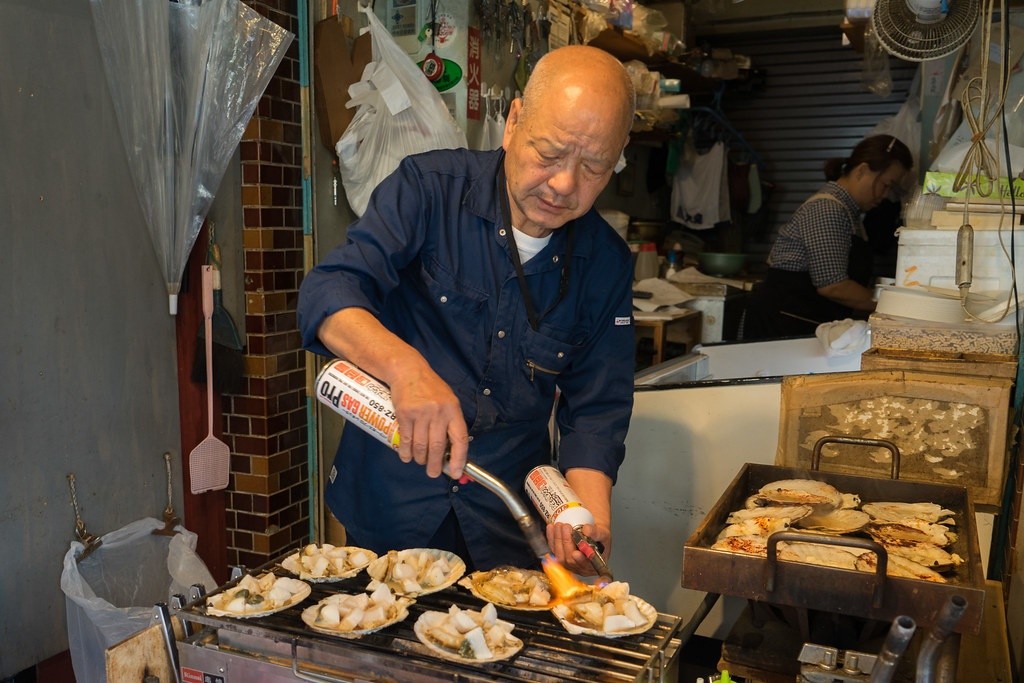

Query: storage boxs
922 171 1024 201
895 230 1024 327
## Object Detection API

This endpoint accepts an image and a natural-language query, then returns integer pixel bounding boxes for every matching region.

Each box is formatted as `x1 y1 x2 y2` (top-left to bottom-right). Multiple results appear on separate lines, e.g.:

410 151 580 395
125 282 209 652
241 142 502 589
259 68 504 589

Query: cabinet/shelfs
574 0 690 142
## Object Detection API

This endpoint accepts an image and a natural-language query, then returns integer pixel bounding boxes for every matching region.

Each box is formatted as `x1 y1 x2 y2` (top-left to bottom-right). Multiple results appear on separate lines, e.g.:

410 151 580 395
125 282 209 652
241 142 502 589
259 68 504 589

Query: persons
763 134 915 342
297 46 637 575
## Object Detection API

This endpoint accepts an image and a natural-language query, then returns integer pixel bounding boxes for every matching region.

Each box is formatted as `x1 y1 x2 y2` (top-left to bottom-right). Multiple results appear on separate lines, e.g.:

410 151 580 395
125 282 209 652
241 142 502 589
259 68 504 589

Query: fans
871 0 984 62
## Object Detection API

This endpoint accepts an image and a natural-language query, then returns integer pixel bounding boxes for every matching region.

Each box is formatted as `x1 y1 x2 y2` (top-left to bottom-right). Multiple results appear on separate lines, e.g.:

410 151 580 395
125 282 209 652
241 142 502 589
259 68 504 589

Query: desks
634 310 703 367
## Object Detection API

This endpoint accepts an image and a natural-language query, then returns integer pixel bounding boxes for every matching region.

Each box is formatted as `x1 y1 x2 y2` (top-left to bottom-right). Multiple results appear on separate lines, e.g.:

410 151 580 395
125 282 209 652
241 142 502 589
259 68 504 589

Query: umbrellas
90 0 298 317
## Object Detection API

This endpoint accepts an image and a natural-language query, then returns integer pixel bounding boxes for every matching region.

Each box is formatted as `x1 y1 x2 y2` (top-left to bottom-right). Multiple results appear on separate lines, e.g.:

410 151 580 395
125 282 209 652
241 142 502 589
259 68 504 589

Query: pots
627 222 665 244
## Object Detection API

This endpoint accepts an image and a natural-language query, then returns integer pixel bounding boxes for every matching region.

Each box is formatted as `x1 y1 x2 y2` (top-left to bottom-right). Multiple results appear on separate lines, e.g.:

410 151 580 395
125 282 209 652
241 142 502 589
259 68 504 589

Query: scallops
205 543 657 664
713 478 964 583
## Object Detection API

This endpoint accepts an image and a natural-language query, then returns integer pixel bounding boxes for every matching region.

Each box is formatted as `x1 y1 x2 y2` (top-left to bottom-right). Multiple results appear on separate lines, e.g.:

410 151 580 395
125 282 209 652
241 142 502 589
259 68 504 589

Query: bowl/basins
698 252 748 275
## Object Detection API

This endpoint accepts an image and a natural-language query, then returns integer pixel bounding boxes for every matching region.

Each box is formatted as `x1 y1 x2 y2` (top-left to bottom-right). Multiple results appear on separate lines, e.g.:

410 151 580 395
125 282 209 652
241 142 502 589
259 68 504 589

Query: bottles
663 251 681 280
634 244 659 282
